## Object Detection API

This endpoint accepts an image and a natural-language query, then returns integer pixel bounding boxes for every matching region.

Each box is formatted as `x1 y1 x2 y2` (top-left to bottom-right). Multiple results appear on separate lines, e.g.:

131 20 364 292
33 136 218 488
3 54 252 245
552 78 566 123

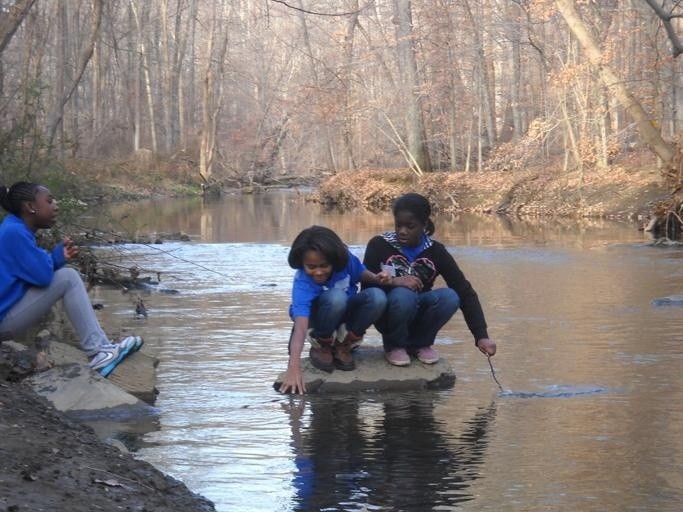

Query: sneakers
383 346 411 366
413 345 440 365
86 334 143 377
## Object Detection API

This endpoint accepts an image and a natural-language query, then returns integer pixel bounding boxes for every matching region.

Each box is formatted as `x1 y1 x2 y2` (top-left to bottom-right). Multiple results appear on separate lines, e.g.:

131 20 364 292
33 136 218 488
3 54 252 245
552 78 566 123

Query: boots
306 328 335 370
336 323 366 371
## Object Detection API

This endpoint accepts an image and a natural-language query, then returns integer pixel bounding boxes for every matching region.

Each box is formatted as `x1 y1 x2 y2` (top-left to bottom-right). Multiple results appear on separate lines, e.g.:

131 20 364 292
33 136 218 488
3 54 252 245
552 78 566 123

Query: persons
278 225 424 394
0 181 143 379
360 194 497 367
